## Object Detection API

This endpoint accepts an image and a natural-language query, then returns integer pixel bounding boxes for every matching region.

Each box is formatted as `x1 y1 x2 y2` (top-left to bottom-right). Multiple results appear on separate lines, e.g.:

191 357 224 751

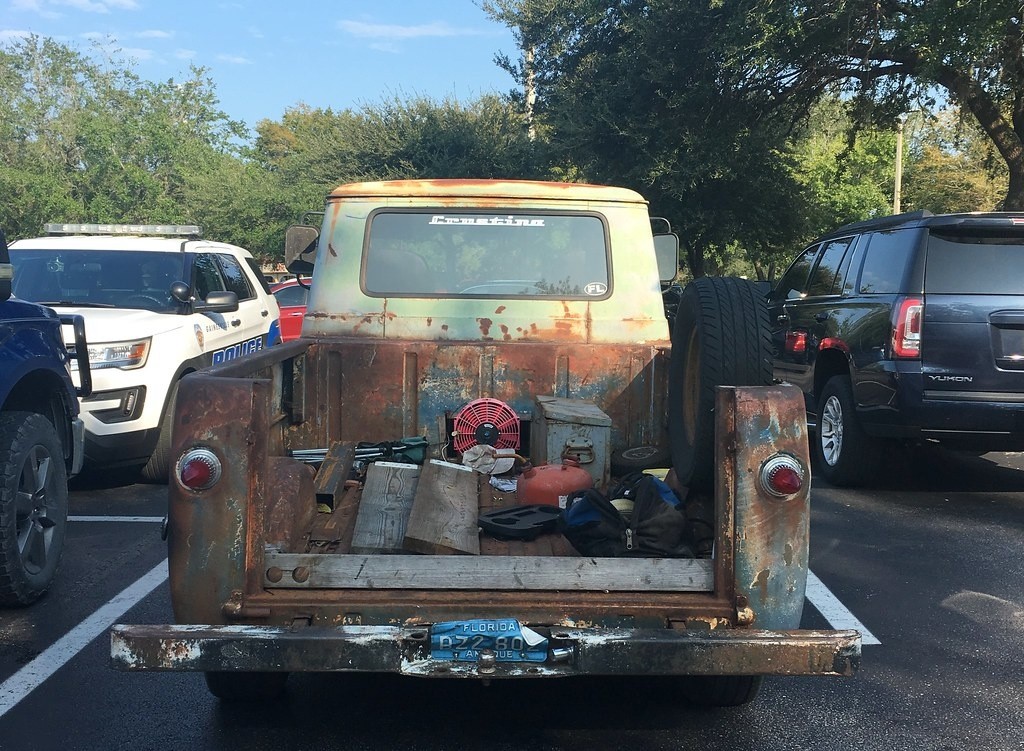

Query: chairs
367 217 431 293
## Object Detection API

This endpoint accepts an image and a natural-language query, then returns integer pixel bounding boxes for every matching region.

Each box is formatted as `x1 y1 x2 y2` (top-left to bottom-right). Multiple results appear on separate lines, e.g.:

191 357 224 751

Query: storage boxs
529 393 613 489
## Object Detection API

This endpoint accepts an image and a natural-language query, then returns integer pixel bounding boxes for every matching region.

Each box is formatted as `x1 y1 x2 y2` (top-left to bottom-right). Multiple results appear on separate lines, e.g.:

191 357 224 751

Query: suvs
5 223 284 484
767 208 1024 488
0 295 92 610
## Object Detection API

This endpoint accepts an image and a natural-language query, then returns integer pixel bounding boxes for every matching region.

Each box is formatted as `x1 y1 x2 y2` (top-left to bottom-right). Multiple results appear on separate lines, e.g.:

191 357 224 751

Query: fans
451 397 522 457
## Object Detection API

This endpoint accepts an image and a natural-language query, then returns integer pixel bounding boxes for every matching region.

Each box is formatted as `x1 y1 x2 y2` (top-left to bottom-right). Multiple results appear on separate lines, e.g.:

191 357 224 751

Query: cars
272 279 311 343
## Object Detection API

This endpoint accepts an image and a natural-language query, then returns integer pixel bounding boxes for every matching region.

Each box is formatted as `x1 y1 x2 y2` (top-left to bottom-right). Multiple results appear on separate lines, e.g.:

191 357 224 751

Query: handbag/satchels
558 472 699 559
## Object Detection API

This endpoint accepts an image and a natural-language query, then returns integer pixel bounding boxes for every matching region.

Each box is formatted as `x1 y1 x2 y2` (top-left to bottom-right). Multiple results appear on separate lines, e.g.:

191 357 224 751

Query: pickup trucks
107 177 883 712
